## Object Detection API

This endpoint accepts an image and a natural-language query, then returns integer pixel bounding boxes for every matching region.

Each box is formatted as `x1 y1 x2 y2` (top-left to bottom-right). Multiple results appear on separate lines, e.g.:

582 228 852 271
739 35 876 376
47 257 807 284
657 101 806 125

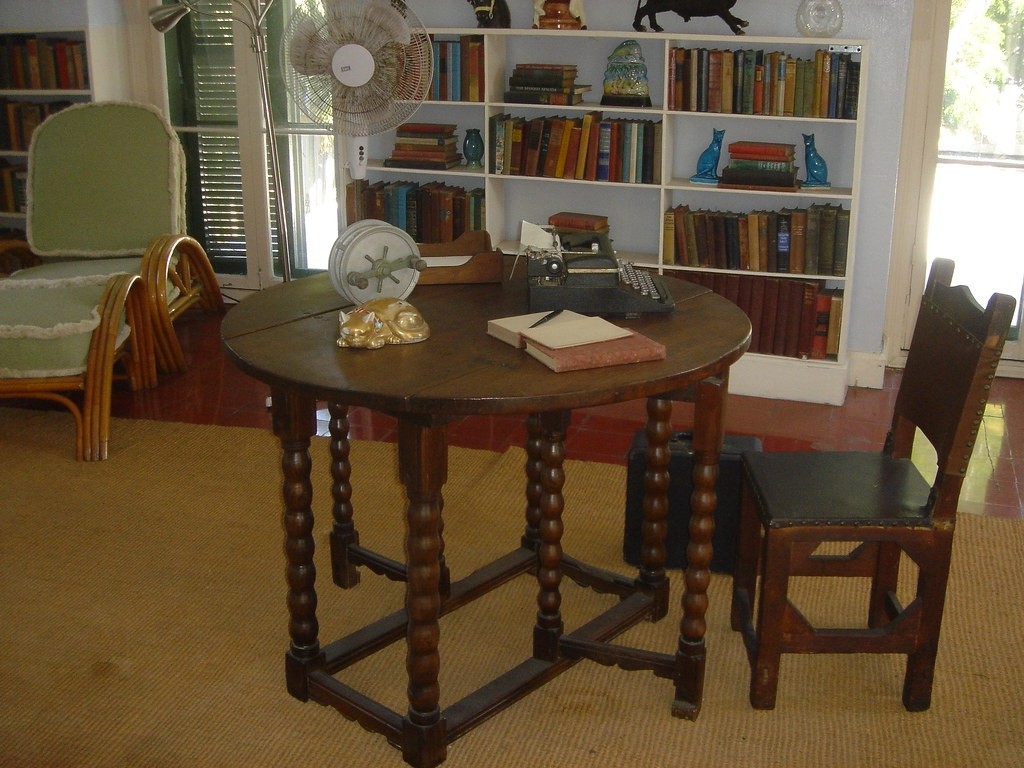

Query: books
520 330 667 373
340 34 664 250
485 307 634 351
0 32 95 279
667 30 863 361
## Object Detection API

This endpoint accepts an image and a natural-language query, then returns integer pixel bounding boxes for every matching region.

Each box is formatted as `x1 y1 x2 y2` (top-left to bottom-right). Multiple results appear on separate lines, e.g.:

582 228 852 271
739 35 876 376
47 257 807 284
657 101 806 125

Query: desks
218 247 757 767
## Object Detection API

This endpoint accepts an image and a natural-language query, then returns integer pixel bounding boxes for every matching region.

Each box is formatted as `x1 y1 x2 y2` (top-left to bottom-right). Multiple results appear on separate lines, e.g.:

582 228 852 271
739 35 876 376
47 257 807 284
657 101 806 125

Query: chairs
728 259 1019 710
3 101 228 462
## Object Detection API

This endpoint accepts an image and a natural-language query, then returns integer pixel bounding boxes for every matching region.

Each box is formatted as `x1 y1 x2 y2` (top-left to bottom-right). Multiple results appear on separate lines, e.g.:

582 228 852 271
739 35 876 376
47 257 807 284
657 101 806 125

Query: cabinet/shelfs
1 31 91 279
335 26 868 408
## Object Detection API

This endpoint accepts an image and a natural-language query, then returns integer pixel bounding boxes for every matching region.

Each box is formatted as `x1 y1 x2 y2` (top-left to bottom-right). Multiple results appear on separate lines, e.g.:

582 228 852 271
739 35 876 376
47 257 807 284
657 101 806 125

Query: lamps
149 1 300 287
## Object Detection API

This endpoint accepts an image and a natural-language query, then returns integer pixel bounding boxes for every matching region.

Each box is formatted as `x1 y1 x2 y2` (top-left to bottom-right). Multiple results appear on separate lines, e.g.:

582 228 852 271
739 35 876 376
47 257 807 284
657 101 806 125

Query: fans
279 0 436 225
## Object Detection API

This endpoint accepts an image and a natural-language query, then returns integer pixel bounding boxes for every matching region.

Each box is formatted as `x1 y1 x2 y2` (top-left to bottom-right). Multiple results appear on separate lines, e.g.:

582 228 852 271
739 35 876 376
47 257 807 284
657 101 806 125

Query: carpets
0 408 1022 768
